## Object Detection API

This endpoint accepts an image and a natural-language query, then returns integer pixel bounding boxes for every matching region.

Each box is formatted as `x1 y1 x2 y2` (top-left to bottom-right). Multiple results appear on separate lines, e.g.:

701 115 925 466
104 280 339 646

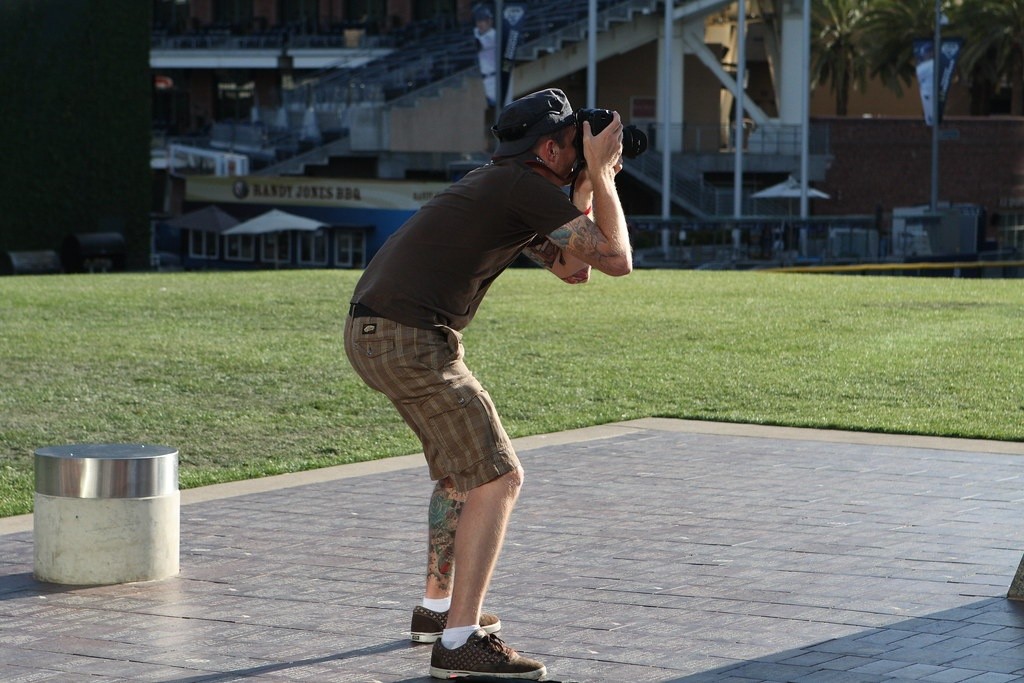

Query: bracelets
583 206 593 216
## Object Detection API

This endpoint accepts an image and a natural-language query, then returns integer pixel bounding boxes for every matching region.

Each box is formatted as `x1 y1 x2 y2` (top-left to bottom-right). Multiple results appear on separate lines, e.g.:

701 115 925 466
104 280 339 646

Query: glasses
491 111 560 141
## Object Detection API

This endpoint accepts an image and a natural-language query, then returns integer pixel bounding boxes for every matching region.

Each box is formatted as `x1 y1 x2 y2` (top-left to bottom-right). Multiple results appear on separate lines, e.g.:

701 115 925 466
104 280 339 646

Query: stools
28 437 182 590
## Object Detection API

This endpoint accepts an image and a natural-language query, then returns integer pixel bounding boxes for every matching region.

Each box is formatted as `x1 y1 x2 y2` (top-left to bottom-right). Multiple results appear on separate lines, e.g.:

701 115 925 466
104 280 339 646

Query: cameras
574 109 647 168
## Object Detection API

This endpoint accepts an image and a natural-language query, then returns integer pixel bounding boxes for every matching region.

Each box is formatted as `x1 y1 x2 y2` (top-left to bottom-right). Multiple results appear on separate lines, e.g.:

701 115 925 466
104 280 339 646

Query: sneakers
411 606 501 643
430 629 546 681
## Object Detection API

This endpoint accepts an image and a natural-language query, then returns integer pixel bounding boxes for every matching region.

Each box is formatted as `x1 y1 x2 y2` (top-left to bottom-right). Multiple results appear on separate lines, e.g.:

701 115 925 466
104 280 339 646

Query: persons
343 89 633 679
474 6 495 151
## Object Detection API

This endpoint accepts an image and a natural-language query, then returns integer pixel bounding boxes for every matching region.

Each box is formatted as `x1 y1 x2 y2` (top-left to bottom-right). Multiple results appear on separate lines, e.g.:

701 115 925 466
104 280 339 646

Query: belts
349 306 380 317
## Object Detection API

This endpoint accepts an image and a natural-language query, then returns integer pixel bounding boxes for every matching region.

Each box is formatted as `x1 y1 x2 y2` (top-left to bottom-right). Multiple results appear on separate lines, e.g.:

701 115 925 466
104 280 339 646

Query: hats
493 87 574 157
